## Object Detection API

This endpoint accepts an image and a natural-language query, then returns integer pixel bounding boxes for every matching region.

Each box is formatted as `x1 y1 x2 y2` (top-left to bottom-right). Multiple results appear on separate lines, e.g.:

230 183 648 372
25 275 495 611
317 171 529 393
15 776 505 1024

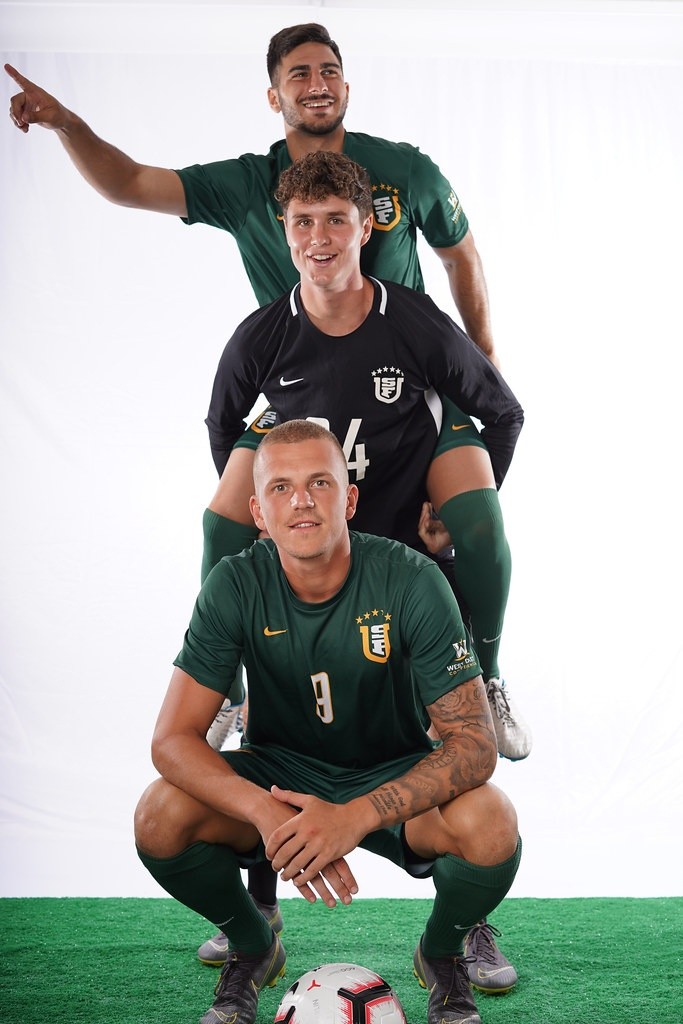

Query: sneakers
462 915 519 995
197 901 284 967
472 667 525 763
201 929 287 1024
402 928 480 1024
204 687 238 751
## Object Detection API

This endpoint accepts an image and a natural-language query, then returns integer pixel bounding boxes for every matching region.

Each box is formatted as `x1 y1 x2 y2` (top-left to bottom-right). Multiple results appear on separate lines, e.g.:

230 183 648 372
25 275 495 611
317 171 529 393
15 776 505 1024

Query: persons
3 22 531 764
198 147 525 969
130 419 524 1023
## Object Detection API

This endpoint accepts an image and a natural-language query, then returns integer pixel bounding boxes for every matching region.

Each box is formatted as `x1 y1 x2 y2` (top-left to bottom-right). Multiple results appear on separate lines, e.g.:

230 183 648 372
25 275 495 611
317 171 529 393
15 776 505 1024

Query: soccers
272 961 408 1024
